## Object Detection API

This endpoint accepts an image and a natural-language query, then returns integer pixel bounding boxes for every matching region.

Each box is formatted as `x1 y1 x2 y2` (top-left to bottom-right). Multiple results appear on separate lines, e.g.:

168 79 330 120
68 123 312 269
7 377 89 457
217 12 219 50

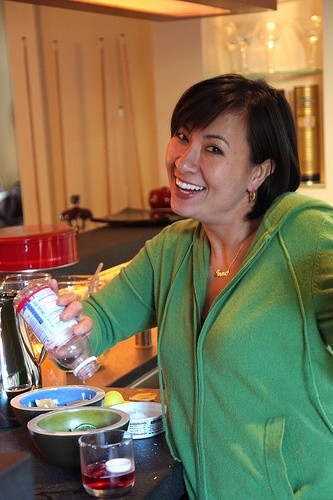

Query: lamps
10 0 278 21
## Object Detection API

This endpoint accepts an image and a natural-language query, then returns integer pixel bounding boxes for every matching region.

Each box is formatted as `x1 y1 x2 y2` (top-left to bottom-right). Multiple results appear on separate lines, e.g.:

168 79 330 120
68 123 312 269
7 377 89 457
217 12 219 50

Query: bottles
13 279 100 381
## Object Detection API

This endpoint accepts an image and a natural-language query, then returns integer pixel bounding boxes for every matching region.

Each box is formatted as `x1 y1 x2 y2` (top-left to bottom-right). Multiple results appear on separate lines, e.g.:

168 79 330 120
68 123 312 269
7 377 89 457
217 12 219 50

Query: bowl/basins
27 406 130 469
10 385 105 457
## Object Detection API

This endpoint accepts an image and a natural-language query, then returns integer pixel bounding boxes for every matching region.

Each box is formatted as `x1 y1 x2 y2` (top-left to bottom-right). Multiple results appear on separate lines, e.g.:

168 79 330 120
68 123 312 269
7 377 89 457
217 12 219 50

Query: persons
47 73 333 500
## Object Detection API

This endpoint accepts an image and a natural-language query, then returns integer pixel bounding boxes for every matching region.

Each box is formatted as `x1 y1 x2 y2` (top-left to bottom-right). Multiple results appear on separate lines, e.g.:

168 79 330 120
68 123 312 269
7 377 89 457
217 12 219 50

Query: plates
91 207 188 225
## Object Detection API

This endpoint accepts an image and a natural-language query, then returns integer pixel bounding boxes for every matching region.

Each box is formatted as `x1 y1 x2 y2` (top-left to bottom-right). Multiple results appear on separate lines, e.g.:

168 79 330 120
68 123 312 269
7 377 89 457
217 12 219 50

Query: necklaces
209 238 245 277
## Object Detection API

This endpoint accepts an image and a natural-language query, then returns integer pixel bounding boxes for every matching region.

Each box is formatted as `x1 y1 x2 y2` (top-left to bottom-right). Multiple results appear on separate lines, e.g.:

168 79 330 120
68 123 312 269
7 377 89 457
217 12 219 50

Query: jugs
0 289 48 428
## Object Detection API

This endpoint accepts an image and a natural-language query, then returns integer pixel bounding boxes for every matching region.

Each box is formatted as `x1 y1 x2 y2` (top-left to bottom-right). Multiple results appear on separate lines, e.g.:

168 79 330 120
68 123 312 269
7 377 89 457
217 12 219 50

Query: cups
79 429 136 497
51 275 109 371
4 273 51 360
0 294 47 399
